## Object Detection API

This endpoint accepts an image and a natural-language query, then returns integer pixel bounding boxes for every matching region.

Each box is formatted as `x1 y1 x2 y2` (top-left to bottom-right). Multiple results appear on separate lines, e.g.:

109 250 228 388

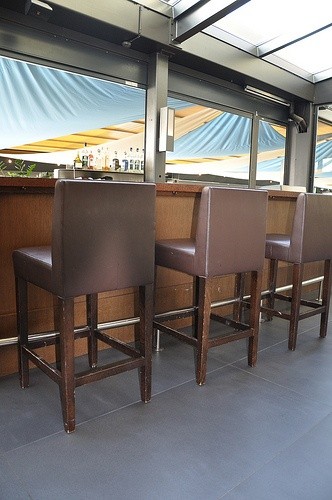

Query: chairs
12 179 332 435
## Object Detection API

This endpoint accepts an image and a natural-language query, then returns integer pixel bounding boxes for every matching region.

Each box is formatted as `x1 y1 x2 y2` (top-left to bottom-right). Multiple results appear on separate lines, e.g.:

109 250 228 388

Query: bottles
74 142 144 172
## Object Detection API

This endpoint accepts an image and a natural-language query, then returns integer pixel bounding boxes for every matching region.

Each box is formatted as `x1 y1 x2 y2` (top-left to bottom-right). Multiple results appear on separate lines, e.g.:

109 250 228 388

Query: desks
0 177 332 378
